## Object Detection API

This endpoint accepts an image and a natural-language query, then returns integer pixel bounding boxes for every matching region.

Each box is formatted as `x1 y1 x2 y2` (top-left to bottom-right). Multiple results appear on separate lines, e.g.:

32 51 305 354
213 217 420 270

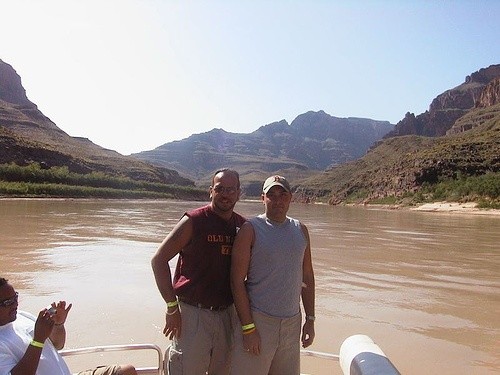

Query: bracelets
241 328 256 335
305 316 315 320
30 339 44 348
166 300 178 307
165 306 179 315
53 323 65 326
241 323 255 331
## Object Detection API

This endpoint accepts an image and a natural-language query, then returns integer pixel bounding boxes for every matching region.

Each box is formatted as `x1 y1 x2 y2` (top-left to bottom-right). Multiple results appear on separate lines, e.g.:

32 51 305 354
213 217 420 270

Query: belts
178 297 216 311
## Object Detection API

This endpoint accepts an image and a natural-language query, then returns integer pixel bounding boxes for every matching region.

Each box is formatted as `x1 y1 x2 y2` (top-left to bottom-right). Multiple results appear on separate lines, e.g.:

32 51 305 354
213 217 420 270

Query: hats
262 175 290 194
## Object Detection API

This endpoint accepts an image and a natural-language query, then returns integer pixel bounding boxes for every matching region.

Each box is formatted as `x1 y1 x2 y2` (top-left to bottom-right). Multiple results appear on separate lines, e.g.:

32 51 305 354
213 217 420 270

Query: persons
0 276 138 375
228 173 316 375
151 169 259 375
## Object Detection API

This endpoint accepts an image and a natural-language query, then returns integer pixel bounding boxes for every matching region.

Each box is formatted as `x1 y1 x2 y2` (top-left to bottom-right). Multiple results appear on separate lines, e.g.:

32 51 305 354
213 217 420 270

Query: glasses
0 292 19 307
212 186 236 193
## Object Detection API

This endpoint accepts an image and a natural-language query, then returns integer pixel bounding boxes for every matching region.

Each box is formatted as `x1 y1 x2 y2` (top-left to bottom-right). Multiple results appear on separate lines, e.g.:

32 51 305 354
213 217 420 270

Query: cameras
48 307 58 315
